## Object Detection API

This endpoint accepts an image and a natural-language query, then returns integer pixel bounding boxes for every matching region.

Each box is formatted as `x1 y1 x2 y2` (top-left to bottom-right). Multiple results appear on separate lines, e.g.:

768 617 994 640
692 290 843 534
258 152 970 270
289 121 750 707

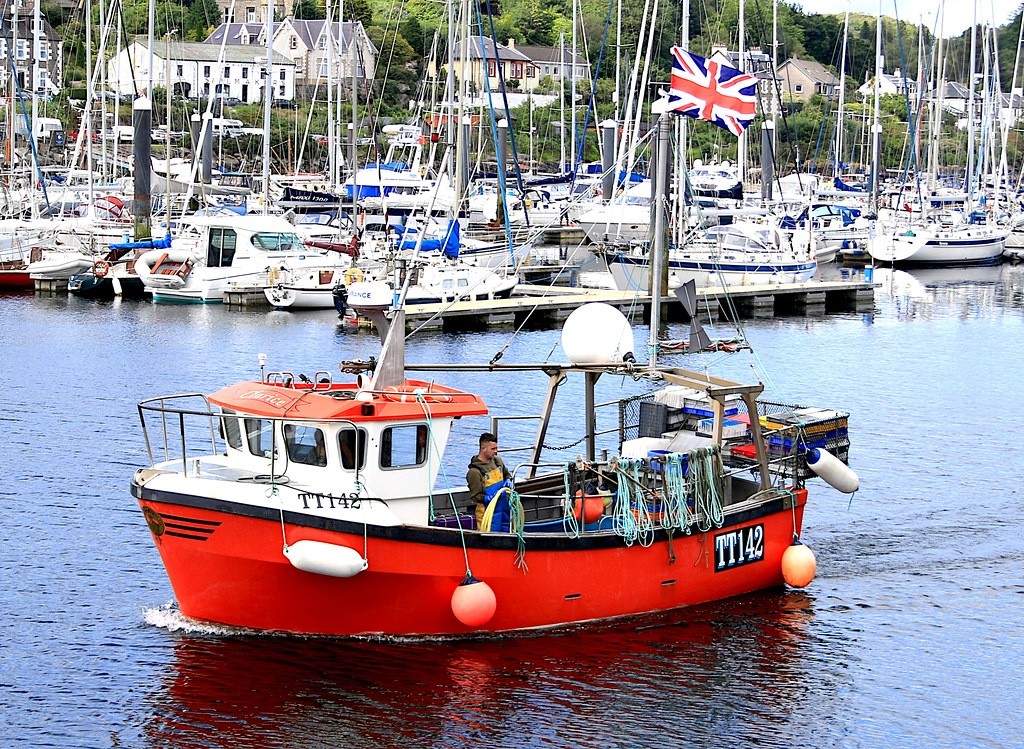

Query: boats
126 117 811 634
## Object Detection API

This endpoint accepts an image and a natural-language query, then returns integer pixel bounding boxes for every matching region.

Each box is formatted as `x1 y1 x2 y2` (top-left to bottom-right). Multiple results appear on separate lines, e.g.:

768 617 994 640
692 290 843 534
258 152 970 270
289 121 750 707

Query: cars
271 98 300 110
122 94 142 100
359 138 371 143
313 136 327 145
16 91 32 101
67 128 96 144
96 91 126 105
96 127 115 140
150 129 167 143
173 95 190 104
226 96 249 107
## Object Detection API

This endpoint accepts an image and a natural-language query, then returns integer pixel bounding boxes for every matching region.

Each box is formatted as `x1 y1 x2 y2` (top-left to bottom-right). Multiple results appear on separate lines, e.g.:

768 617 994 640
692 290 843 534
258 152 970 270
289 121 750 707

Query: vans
114 126 134 144
36 117 61 141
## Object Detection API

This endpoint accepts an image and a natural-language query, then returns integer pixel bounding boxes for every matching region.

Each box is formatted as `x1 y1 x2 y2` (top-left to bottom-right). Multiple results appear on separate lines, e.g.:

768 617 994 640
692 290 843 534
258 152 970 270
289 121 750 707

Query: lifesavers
345 268 363 289
270 267 279 286
383 384 453 404
93 260 109 278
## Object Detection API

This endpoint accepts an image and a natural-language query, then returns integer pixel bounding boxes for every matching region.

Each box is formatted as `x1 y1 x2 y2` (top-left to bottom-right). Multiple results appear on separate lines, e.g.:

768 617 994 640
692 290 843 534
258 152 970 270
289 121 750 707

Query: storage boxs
648 450 692 477
698 417 747 439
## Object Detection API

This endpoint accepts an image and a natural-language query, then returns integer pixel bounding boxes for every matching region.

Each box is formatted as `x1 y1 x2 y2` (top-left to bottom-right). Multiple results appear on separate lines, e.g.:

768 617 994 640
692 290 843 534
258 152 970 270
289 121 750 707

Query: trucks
212 118 244 138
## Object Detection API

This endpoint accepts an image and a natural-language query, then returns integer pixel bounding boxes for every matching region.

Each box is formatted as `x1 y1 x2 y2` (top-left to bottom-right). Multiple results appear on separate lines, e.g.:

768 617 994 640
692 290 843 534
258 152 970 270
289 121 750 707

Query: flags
669 47 760 137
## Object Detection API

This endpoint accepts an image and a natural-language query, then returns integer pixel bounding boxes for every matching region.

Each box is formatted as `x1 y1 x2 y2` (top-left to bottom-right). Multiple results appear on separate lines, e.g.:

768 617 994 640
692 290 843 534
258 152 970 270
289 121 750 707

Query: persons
466 433 514 532
559 197 569 226
314 431 352 468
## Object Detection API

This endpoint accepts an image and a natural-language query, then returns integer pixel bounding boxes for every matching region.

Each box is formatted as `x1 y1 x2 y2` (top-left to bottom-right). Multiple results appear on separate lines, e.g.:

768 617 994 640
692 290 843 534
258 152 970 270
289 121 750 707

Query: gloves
484 491 498 502
503 479 515 489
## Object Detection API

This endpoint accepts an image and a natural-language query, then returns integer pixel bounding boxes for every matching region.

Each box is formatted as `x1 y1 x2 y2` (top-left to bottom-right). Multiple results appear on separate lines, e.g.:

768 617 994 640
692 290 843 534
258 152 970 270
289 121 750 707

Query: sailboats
0 0 1024 309
